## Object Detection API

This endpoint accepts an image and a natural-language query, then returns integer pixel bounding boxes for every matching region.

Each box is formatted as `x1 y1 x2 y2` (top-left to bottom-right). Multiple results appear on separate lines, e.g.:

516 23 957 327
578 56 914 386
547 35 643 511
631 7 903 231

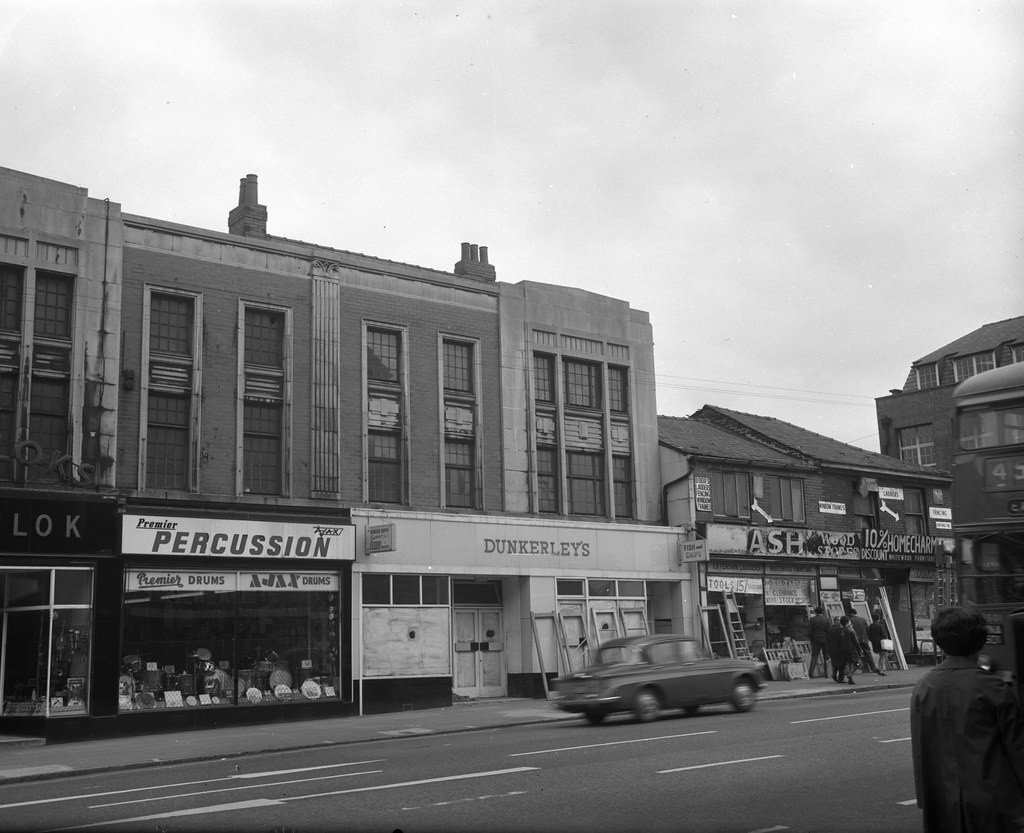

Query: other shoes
848 678 856 684
832 674 838 682
825 675 828 678
809 673 816 678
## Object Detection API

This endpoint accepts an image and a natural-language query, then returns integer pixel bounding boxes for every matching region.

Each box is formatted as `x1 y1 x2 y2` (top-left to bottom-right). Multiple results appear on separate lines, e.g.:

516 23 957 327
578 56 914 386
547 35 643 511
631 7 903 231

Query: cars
556 634 763 722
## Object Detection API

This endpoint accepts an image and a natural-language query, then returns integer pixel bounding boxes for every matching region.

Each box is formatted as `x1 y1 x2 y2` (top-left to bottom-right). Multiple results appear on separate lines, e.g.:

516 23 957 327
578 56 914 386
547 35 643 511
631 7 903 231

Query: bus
950 360 1024 684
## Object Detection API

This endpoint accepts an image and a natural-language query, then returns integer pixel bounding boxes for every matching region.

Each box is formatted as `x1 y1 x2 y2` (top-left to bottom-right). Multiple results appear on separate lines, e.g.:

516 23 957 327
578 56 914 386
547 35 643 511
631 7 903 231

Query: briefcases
881 639 893 650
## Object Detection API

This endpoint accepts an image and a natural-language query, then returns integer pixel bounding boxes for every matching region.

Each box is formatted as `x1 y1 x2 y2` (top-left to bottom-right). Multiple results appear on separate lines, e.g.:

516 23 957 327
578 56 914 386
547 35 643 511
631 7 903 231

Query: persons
849 609 878 672
808 607 831 678
828 616 863 684
910 606 1024 833
866 613 888 676
